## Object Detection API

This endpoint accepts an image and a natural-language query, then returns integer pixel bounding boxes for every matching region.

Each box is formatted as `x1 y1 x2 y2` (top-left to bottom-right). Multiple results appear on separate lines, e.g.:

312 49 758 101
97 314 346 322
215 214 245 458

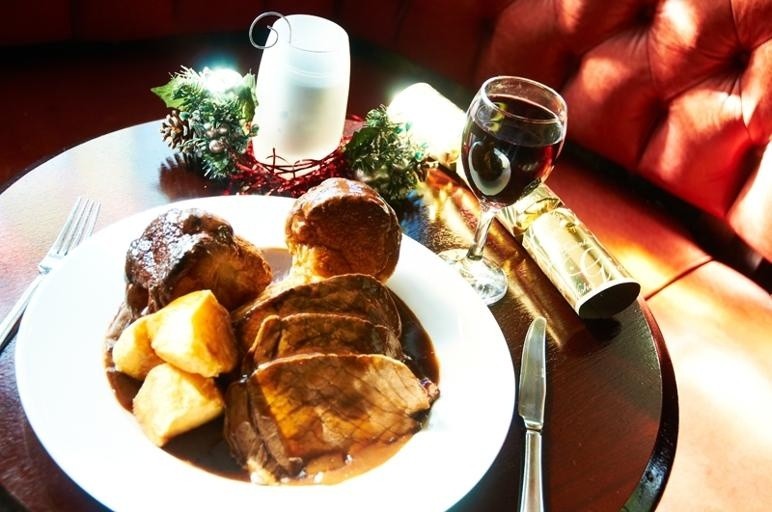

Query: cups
252 14 351 181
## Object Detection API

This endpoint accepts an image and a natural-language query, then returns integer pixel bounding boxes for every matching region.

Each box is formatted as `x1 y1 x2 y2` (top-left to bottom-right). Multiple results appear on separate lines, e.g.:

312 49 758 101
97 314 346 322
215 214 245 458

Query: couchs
445 1 772 512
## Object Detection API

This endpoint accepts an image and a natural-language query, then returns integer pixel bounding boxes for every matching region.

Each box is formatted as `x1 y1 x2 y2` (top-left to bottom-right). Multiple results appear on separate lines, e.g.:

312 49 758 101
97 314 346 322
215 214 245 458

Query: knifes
517 316 548 512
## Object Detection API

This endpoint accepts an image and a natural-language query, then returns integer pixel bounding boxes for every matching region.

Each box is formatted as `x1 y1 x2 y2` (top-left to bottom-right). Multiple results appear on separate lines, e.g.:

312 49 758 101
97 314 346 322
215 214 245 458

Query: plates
16 194 516 512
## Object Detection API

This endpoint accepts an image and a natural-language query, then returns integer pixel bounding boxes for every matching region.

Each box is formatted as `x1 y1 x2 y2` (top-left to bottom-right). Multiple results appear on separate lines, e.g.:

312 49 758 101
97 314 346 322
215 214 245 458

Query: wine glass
437 75 568 307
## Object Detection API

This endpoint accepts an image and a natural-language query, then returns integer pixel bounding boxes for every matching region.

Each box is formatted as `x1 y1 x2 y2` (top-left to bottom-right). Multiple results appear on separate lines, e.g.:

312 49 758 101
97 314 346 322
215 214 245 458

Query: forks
0 195 101 354
